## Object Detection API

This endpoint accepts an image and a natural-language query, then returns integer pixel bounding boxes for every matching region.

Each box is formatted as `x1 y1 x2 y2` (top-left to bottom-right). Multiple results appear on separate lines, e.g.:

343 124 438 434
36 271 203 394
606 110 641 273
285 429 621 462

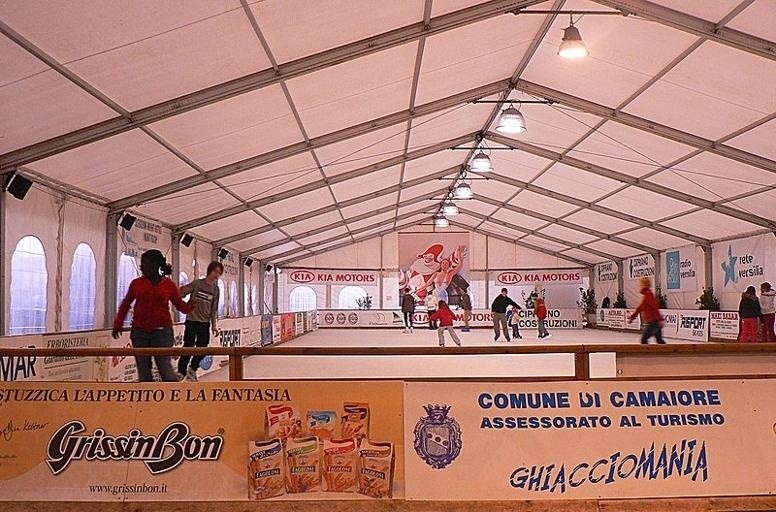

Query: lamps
432 13 588 230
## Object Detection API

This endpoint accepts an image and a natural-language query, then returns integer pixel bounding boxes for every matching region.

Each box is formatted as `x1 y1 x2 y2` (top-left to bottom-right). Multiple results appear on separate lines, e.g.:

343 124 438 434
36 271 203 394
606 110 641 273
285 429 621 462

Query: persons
177 261 224 381
627 277 666 344
461 288 472 331
427 300 461 347
401 288 415 333
425 291 438 330
760 282 776 343
491 287 549 342
111 249 197 382
415 246 466 305
738 286 766 343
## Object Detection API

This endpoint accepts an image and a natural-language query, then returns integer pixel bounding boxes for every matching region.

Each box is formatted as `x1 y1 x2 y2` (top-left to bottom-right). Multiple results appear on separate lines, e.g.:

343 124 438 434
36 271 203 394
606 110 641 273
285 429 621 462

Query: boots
410 326 414 334
403 327 410 334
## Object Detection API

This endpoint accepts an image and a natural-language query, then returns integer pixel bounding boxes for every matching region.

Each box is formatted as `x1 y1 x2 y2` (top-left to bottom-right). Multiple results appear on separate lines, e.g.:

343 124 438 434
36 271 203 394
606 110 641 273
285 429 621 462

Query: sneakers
505 335 510 341
176 370 186 383
186 365 200 382
513 335 522 339
494 334 499 341
543 333 549 337
538 335 543 338
461 327 469 332
